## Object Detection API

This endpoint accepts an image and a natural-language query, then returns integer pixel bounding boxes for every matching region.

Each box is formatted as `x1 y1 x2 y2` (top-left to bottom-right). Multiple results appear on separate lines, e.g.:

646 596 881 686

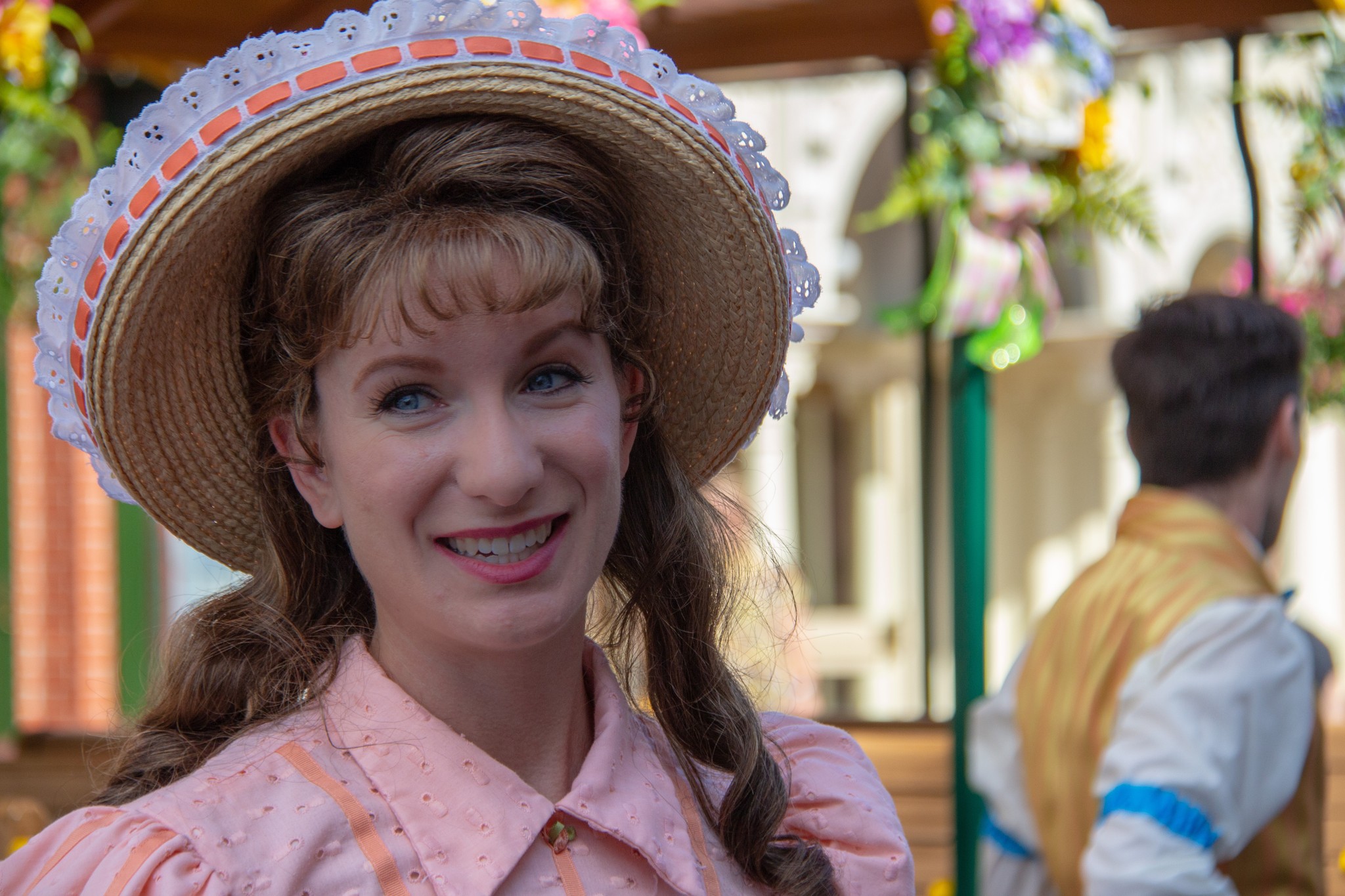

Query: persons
966 291 1333 896
0 0 917 895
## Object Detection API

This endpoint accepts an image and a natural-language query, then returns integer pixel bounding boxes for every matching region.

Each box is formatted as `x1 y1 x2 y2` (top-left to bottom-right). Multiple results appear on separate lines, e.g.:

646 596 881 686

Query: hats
28 0 823 576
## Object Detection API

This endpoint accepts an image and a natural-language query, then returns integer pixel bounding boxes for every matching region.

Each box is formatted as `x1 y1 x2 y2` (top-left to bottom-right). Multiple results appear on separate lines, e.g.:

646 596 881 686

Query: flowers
1218 0 1345 413
856 1 1153 373
